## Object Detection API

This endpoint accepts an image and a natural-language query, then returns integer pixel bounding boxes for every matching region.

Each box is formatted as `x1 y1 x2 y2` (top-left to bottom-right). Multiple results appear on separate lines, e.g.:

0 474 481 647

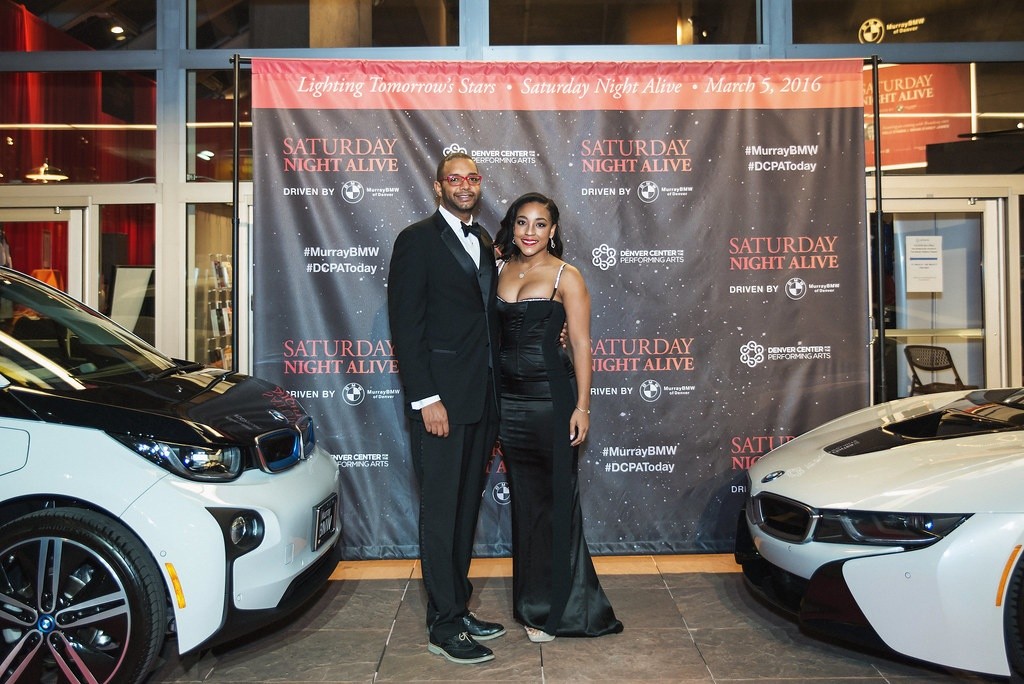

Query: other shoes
525 626 556 643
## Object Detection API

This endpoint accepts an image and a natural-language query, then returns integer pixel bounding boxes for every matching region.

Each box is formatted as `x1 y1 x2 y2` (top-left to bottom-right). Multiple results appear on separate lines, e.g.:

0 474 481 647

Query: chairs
904 344 978 396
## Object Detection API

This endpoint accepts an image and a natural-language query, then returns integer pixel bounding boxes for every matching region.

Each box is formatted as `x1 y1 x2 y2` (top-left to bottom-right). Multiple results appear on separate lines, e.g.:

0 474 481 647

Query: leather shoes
428 632 495 664
460 609 505 640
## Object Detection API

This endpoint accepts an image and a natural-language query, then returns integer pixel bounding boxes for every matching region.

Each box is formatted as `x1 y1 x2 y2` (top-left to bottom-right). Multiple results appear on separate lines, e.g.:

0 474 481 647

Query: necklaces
516 252 549 279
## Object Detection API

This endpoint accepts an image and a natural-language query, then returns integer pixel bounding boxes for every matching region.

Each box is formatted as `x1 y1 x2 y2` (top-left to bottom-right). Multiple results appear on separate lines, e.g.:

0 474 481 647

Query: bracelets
577 406 590 415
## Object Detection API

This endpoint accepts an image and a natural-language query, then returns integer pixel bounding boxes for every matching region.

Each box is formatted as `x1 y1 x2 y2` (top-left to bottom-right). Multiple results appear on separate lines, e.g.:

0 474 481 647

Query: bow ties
460 221 482 239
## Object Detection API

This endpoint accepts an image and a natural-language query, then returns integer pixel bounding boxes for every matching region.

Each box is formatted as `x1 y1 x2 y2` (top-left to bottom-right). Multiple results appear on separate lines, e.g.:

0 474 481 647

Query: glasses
439 173 482 186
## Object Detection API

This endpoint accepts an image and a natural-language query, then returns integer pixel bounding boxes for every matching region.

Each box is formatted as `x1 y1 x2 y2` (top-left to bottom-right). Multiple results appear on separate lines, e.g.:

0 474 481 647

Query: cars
732 386 1024 682
0 263 343 684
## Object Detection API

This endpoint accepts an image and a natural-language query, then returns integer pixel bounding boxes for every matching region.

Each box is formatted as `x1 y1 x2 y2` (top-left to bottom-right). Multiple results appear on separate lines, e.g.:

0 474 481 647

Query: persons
388 152 567 664
494 191 624 642
